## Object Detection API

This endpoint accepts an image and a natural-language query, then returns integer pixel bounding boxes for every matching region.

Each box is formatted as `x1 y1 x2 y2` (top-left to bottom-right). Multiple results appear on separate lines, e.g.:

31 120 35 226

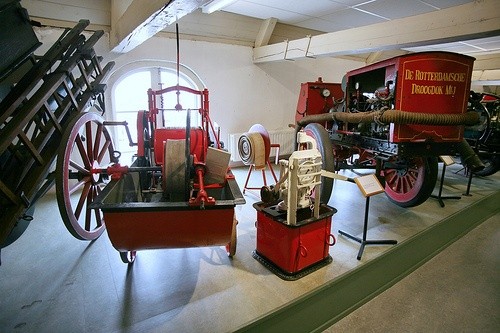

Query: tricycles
88 81 249 264
293 49 476 209
